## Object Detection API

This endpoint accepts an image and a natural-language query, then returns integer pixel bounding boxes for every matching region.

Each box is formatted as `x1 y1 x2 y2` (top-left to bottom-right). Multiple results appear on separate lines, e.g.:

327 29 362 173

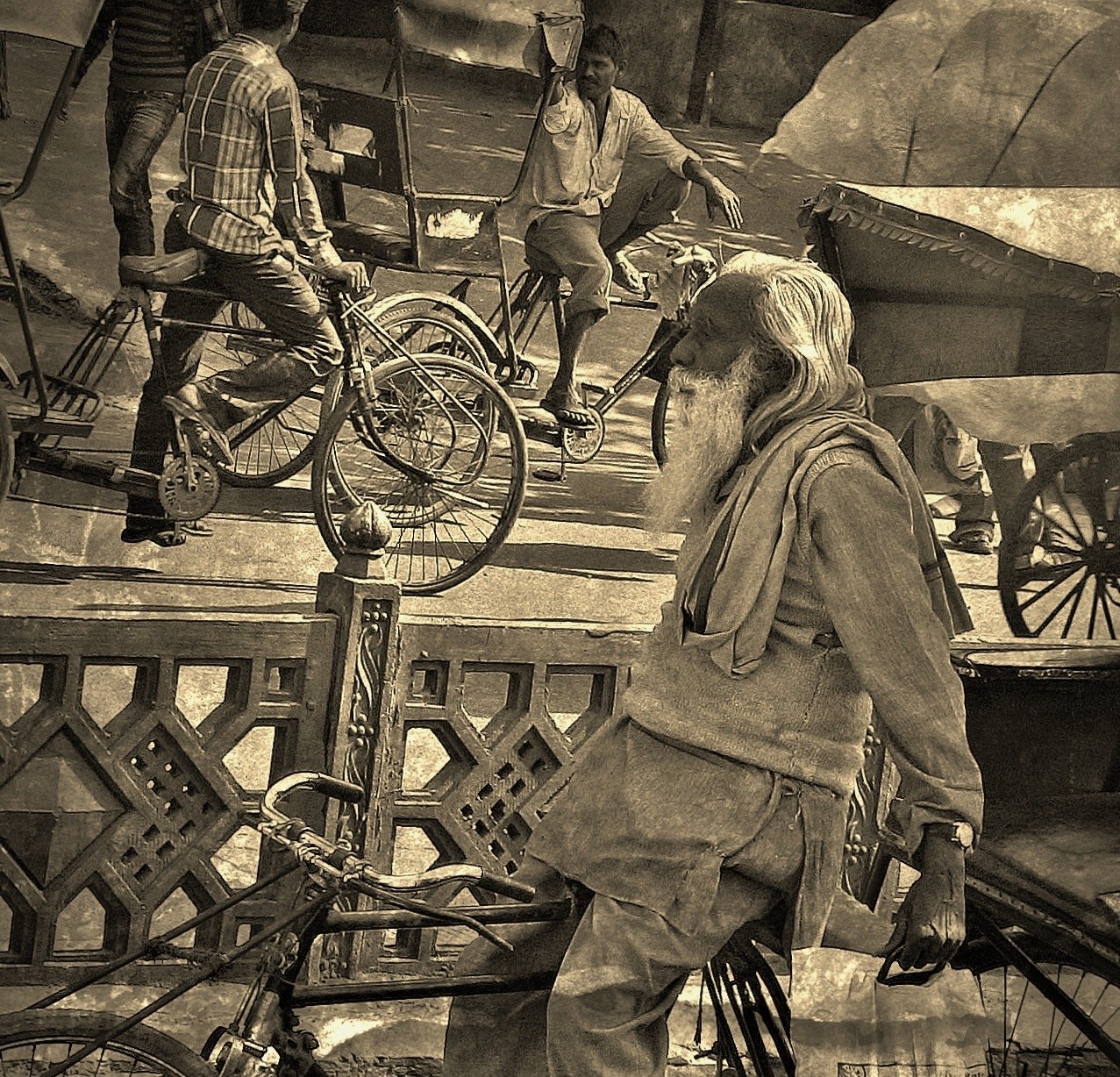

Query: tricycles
0 0 730 601
0 634 1120 1077
796 174 1120 646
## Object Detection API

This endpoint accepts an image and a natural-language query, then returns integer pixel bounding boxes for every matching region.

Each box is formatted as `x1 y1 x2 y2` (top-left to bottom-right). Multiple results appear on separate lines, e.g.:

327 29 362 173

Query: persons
515 24 743 428
120 0 372 547
443 251 984 1077
928 408 1079 586
56 0 231 323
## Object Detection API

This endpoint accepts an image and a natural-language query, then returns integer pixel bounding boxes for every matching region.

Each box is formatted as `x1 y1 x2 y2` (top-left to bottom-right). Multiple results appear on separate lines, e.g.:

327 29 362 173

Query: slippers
610 259 647 294
1042 548 1078 566
161 396 235 466
121 527 186 547
958 532 994 554
540 399 597 431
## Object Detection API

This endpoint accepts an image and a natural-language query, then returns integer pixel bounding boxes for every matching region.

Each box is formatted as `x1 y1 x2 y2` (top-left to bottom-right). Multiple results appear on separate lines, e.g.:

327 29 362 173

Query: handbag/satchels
791 947 993 1077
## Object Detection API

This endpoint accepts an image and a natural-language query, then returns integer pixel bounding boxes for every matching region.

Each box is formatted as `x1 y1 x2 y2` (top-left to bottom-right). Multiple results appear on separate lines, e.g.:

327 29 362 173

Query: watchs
925 821 973 851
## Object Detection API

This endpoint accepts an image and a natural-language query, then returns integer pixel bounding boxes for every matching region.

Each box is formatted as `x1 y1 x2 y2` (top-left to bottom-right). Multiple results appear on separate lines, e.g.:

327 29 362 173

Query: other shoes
97 292 164 322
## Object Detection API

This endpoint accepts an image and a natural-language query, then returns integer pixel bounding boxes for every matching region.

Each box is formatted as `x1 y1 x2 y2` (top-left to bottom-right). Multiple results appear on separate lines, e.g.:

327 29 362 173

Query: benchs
298 78 501 278
866 641 1120 952
